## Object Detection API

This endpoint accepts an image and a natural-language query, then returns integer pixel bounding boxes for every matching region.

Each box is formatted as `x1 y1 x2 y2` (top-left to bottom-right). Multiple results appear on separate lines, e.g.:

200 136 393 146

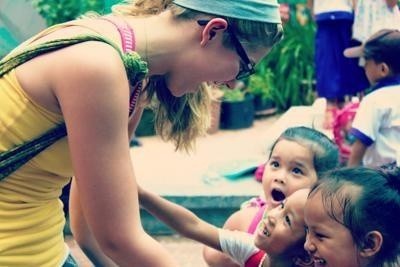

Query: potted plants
219 64 276 130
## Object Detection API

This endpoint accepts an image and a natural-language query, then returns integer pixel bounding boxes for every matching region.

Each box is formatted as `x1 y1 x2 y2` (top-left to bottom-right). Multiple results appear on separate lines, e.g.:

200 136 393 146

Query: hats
173 0 282 24
343 28 399 58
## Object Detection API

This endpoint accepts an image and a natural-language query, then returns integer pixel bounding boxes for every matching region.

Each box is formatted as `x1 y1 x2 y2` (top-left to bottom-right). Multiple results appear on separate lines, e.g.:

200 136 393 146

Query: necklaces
144 15 150 75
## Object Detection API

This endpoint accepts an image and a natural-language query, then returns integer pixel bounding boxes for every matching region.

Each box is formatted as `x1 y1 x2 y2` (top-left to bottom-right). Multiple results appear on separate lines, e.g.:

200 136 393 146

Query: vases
135 105 158 137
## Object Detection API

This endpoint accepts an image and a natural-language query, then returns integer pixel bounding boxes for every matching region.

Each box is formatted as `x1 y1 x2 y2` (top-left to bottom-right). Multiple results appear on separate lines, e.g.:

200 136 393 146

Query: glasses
197 20 255 80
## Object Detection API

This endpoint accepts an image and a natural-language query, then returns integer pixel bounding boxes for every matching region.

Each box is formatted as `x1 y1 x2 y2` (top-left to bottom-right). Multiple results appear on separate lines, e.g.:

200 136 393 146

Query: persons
344 29 399 170
351 0 399 69
302 161 400 266
133 180 316 267
204 126 343 266
314 0 368 129
2 0 284 266
